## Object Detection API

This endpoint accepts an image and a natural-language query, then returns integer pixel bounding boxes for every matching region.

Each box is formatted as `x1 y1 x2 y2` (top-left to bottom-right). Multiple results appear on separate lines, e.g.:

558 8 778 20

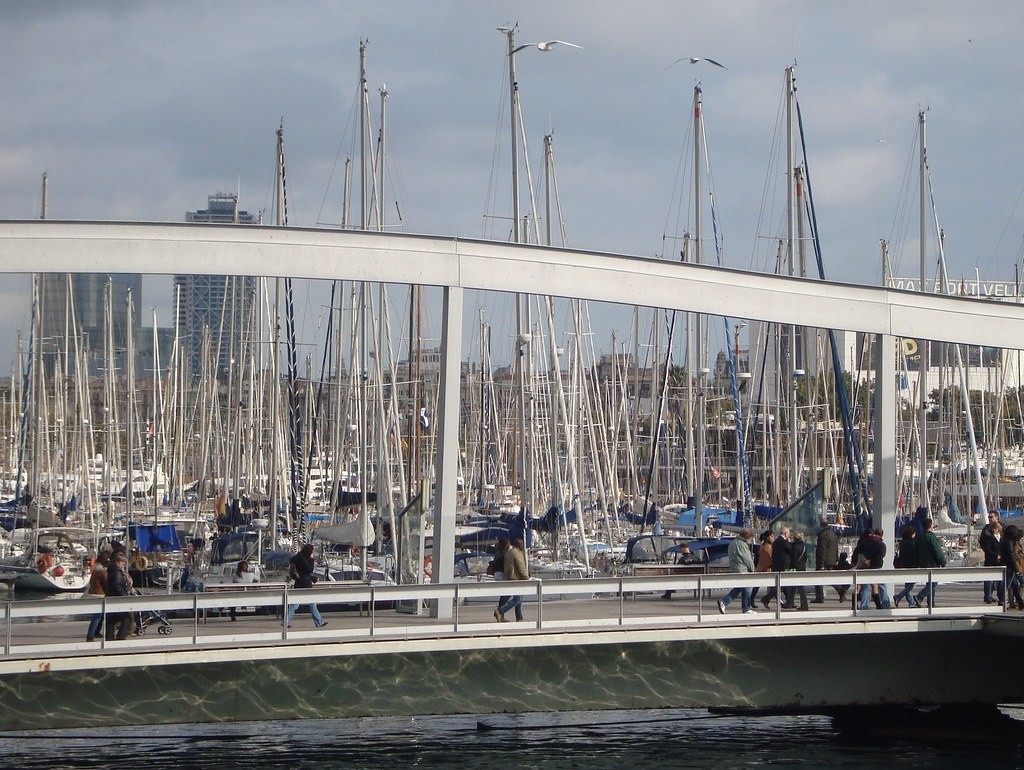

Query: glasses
988 516 995 518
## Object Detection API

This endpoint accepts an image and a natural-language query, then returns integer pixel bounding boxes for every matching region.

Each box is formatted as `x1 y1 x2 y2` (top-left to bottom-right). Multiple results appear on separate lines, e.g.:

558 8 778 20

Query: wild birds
663 56 730 71
505 40 585 57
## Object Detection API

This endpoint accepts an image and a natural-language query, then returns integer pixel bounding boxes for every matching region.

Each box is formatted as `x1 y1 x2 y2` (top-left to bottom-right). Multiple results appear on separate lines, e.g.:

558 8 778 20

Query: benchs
624 563 757 602
202 582 371 623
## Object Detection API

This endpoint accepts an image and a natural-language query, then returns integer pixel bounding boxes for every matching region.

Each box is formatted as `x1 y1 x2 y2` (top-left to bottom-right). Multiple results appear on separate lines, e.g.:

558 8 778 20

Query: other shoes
500 613 509 622
230 613 236 621
318 621 329 627
95 633 103 638
661 594 671 600
517 618 526 622
87 637 93 642
281 623 292 628
494 610 502 623
717 590 1006 614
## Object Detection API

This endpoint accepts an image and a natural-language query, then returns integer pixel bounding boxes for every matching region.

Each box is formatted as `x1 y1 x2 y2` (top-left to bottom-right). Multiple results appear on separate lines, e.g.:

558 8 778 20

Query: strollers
128 583 174 636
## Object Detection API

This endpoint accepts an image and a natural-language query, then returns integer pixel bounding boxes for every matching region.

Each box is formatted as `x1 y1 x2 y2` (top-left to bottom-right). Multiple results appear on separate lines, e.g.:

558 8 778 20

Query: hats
680 543 689 548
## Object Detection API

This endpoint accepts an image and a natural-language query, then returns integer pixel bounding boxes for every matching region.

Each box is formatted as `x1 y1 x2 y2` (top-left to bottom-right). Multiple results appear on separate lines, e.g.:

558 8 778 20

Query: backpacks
290 559 300 580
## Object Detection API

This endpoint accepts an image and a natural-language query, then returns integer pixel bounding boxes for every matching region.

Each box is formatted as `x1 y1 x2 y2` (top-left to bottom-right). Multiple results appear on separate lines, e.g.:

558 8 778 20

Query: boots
1008 589 1017 608
1015 587 1024 610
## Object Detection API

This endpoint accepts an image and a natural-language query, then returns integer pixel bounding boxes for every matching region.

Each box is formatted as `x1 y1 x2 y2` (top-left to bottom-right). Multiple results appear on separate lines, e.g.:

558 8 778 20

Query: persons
283 544 329 629
86 546 137 641
716 529 758 614
834 551 853 590
658 542 702 600
892 526 922 608
750 526 810 611
810 519 848 603
913 518 946 608
486 536 528 622
977 509 1024 610
850 528 890 610
218 561 260 621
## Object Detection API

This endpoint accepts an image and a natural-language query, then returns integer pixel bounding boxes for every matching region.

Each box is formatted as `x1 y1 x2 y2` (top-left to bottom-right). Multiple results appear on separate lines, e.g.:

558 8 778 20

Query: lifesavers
424 556 433 576
37 558 47 574
135 556 148 572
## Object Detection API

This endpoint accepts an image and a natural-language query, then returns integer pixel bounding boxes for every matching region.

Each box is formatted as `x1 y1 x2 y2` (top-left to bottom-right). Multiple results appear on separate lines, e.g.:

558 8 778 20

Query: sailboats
0 23 1023 594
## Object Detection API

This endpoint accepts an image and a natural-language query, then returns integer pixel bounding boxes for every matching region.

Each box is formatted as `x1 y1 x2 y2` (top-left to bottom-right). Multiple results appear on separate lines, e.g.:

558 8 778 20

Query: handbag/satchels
894 554 902 569
487 561 496 575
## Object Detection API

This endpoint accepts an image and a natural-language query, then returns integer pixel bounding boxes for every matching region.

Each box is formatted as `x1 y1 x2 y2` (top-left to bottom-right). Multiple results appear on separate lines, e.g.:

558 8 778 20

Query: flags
215 490 226 514
706 453 720 480
392 436 408 448
419 401 429 430
